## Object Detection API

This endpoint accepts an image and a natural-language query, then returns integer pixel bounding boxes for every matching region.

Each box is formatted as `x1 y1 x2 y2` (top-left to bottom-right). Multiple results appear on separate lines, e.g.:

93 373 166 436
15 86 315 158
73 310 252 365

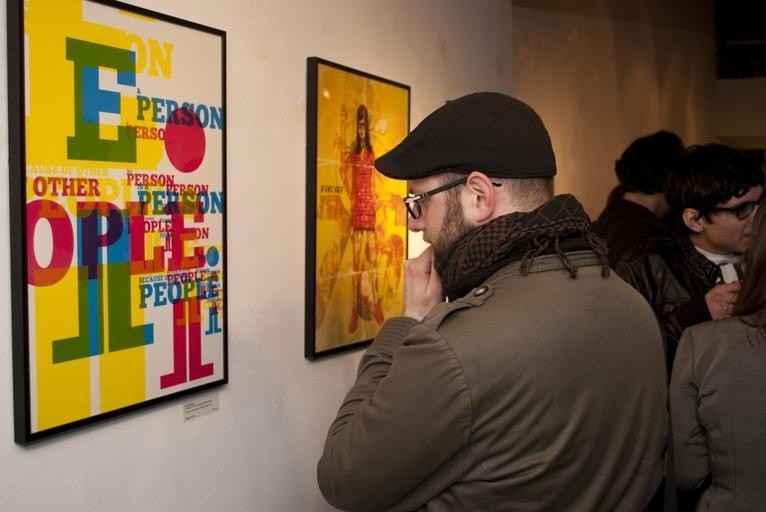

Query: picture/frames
304 56 409 362
7 0 226 447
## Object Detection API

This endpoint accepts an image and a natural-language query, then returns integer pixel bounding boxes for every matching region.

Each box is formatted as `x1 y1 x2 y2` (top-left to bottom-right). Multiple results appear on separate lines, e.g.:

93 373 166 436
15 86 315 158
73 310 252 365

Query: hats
374 91 558 179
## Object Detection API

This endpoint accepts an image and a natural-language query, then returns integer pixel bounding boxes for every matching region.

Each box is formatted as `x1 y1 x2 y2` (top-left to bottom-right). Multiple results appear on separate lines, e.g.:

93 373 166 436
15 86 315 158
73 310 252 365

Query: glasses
710 189 766 220
403 178 503 220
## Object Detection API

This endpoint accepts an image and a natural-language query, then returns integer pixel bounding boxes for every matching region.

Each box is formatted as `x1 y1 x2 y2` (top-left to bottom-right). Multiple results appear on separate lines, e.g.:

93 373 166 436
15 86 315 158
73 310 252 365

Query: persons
341 105 388 334
665 184 766 512
591 131 694 269
612 142 764 375
318 93 666 512
